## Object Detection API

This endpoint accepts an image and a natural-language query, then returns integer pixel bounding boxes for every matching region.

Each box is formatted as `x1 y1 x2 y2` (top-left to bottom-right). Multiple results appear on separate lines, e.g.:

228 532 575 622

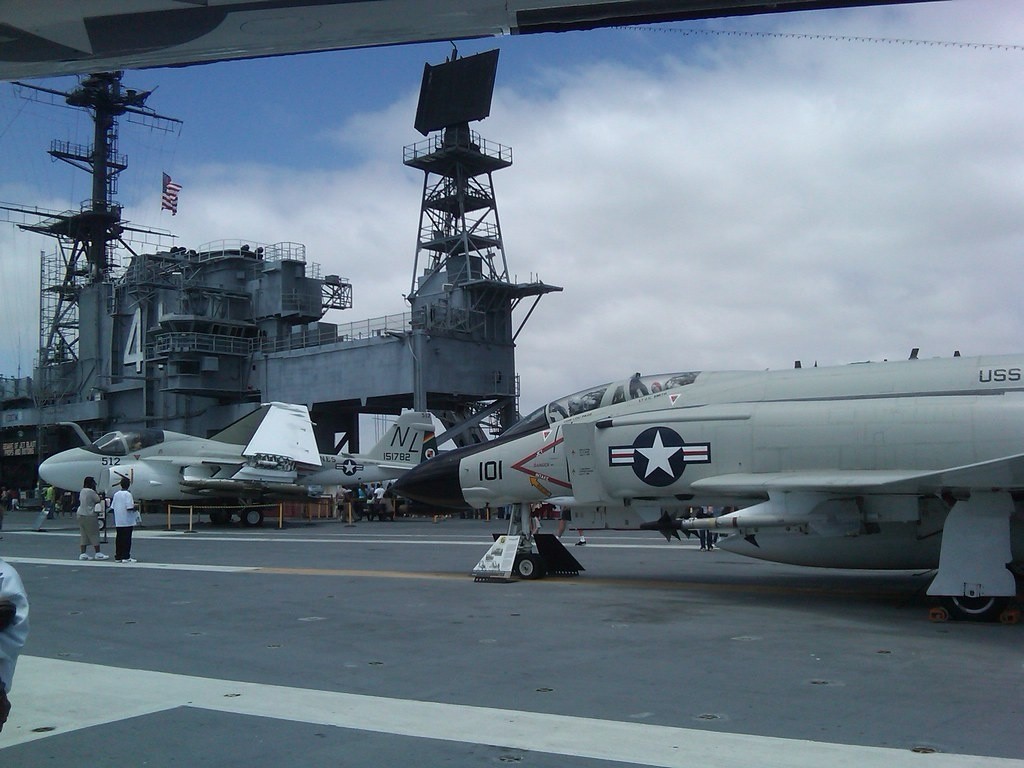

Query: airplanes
38 401 458 527
389 348 1024 621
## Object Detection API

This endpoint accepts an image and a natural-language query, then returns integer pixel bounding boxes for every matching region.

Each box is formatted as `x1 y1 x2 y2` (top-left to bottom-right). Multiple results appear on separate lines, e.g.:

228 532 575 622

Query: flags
161 172 183 216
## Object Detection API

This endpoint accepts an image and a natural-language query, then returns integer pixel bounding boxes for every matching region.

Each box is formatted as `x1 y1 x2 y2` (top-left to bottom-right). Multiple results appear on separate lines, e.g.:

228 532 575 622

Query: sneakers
80 554 92 560
95 554 109 560
122 558 137 562
115 559 121 562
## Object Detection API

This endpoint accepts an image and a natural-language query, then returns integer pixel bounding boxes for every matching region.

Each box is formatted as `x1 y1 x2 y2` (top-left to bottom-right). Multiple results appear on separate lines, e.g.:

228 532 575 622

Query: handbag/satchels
134 510 142 523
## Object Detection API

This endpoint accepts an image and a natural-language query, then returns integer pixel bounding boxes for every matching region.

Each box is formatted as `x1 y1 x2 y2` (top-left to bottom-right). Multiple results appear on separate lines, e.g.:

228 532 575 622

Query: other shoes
709 547 712 550
575 541 586 546
701 547 706 551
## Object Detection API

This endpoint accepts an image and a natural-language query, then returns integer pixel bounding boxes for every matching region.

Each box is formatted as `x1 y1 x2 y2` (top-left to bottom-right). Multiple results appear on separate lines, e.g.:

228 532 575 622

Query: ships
0 39 562 496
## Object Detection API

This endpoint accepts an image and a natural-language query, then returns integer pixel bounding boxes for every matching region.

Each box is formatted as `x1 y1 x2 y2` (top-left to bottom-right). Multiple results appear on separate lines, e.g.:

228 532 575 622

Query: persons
111 478 139 563
528 502 586 546
0 487 22 512
77 477 110 560
568 397 585 416
0 556 30 731
697 506 742 552
337 482 395 523
41 486 74 519
